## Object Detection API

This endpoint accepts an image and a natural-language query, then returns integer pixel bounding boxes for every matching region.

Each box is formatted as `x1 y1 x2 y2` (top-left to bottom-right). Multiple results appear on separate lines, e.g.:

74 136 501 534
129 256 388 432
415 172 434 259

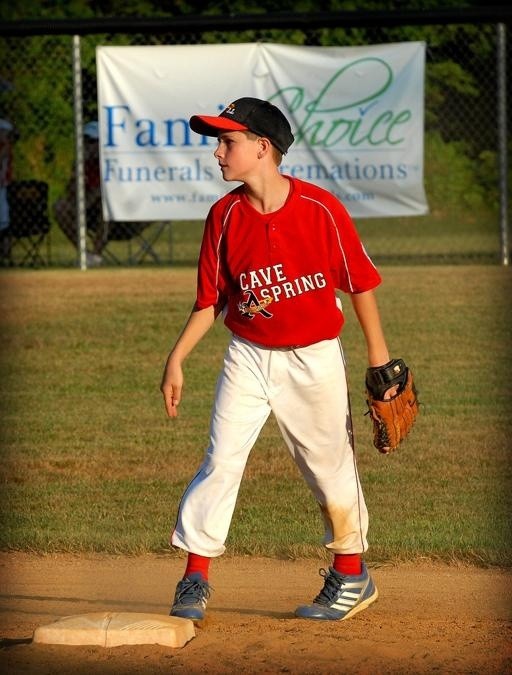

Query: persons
159 96 420 629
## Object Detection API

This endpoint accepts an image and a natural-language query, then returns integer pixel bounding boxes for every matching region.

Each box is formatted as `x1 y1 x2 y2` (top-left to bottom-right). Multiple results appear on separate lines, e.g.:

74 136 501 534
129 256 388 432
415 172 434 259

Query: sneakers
295 559 380 620
169 572 210 620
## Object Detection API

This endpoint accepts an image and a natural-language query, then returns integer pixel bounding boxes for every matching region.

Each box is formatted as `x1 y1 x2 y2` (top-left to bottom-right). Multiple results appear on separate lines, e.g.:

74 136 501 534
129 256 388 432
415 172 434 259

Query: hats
190 97 295 153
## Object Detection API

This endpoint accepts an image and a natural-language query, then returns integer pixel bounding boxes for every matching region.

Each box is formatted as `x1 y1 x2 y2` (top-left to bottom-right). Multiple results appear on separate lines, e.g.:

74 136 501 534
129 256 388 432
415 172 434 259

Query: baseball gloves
366 359 419 454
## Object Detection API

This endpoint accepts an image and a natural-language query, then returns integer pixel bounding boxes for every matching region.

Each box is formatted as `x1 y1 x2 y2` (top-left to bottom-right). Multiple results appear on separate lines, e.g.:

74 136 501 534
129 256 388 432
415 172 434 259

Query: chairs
2 180 172 268
